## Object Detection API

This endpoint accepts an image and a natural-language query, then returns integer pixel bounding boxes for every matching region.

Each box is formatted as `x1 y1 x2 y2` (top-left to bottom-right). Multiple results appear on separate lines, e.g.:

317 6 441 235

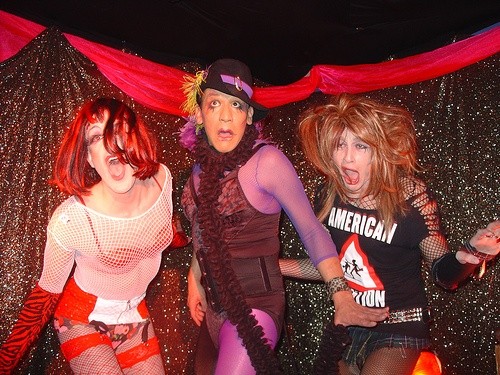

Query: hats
199 58 270 121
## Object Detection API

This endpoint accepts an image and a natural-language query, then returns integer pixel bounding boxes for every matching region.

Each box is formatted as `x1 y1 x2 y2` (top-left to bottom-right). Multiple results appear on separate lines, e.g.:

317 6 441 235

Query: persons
178 59 389 375
0 98 194 375
279 92 500 375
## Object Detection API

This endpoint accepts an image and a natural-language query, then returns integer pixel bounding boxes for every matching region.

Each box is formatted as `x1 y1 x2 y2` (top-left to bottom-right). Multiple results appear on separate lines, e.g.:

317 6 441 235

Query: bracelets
324 276 353 300
